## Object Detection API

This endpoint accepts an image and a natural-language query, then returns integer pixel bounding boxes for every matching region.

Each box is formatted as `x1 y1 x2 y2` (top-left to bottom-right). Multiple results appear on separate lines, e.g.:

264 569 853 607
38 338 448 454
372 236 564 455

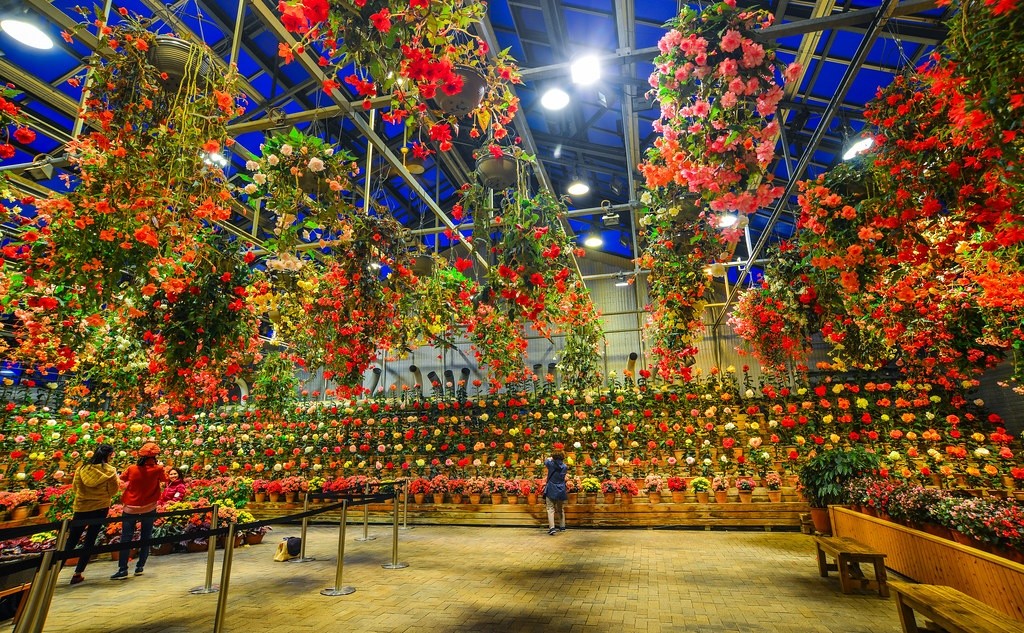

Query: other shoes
110 570 129 579
559 526 565 532
548 528 557 534
134 568 143 575
70 575 84 584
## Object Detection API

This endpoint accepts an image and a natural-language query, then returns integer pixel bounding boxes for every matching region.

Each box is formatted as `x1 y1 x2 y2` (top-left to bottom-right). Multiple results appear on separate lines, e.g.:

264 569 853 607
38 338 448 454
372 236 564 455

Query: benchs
813 536 1024 633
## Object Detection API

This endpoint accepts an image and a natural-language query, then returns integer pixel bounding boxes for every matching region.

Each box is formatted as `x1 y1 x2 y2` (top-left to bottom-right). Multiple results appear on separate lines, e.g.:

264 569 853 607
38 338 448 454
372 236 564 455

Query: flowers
0 480 273 549
0 1 1024 495
842 475 1024 553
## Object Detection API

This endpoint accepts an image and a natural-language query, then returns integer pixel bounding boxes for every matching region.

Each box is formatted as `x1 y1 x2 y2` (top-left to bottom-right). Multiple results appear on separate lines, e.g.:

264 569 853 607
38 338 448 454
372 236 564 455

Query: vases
293 168 332 195
474 153 516 190
277 272 292 287
11 503 265 566
146 38 215 94
250 405 809 504
433 66 487 117
410 256 431 277
850 503 1023 565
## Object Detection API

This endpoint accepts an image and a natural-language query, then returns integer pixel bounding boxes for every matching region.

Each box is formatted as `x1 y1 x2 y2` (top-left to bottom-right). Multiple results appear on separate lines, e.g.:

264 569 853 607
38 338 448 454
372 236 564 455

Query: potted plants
797 446 880 532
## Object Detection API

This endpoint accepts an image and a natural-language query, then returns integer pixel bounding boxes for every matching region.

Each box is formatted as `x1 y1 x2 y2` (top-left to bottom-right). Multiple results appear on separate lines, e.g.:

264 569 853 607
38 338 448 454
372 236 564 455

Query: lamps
619 235 628 248
25 153 55 180
610 174 622 195
600 200 620 227
836 115 873 160
594 86 619 107
632 92 654 111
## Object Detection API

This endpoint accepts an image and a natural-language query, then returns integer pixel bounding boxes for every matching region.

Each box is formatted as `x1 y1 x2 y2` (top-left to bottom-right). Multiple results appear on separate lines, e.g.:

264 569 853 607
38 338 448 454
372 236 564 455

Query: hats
139 442 160 457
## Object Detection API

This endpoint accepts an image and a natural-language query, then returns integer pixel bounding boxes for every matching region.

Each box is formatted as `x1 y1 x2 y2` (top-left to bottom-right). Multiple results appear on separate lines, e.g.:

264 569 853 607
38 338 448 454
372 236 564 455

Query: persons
110 443 167 579
163 467 186 502
59 443 119 583
544 450 567 534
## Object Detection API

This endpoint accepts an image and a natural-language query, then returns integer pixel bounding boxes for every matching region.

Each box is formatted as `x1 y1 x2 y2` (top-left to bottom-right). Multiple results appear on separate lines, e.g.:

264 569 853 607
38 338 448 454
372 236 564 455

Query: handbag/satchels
543 487 547 500
